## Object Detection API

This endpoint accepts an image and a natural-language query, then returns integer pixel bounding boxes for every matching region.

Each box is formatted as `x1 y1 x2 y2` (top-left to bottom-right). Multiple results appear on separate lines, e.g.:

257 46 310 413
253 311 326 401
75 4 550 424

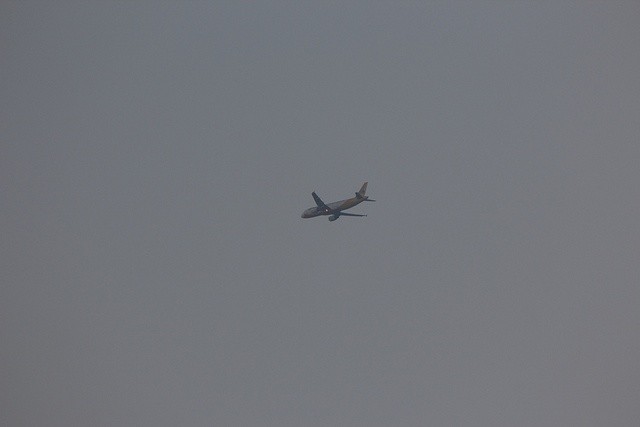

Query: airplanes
300 181 377 221
